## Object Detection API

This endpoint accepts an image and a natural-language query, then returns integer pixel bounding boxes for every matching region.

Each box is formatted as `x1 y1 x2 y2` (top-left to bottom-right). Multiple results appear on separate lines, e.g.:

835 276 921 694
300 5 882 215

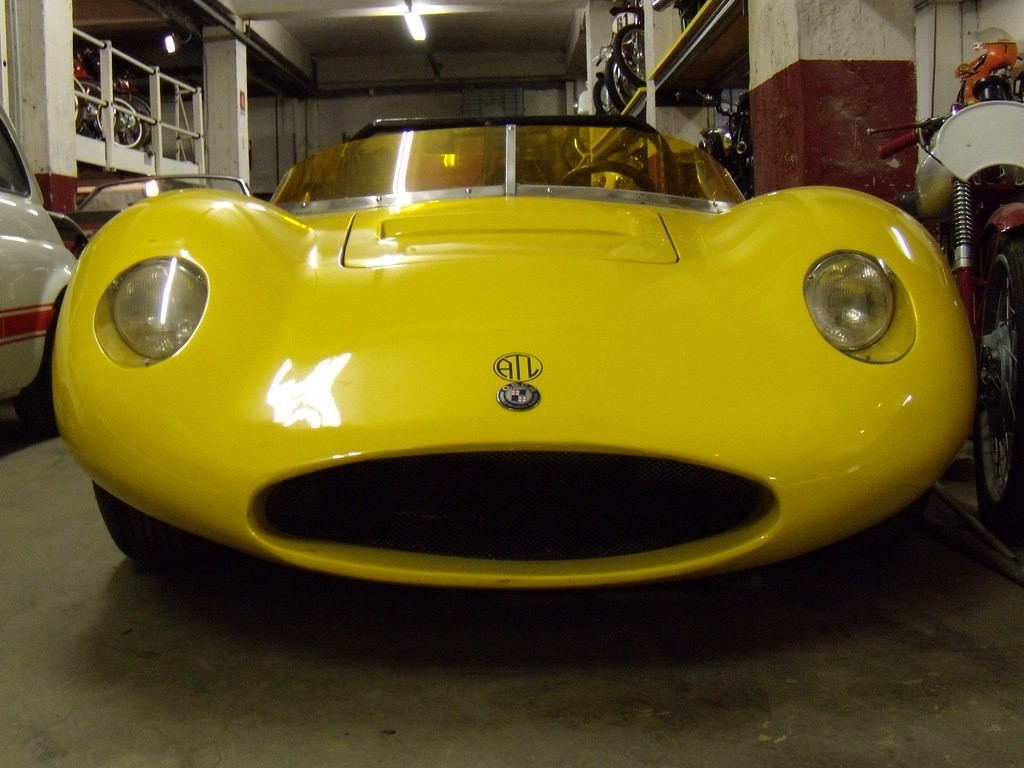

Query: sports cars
53 116 979 589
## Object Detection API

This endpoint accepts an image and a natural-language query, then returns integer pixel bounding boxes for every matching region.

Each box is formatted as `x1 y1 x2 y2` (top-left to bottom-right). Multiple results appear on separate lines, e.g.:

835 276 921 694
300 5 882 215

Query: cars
0 103 88 438
63 175 251 259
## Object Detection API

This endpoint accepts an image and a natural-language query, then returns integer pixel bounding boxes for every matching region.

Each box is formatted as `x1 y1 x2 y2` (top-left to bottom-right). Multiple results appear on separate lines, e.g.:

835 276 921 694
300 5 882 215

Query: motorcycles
73 48 152 150
866 28 1023 588
674 81 755 201
593 1 646 116
